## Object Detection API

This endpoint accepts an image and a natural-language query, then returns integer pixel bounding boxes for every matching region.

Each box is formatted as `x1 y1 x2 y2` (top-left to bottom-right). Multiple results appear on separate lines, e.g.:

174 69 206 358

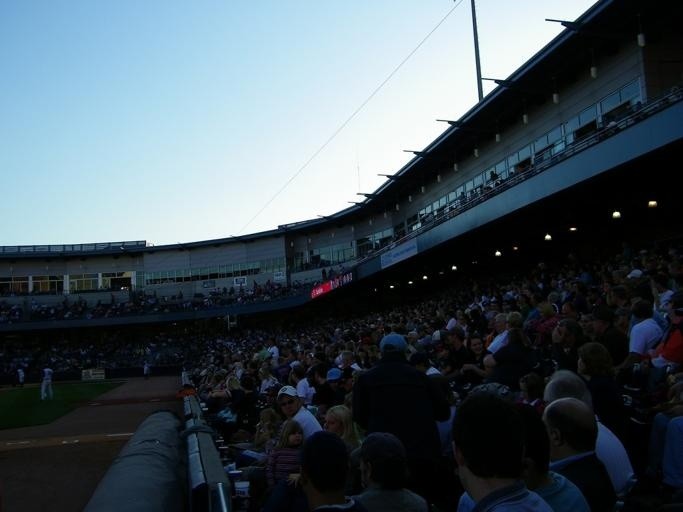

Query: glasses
280 399 294 406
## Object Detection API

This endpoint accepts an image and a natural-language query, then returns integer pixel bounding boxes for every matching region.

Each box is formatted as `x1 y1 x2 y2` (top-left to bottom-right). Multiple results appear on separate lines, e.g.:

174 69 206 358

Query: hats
326 368 342 381
350 431 406 475
380 333 408 353
626 269 642 279
276 385 298 401
264 382 283 392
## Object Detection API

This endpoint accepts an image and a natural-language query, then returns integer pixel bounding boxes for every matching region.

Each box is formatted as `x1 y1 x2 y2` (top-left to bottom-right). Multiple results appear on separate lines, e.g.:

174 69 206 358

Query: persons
416 82 682 222
11 358 28 388
188 221 682 511
0 285 189 381
39 362 54 401
